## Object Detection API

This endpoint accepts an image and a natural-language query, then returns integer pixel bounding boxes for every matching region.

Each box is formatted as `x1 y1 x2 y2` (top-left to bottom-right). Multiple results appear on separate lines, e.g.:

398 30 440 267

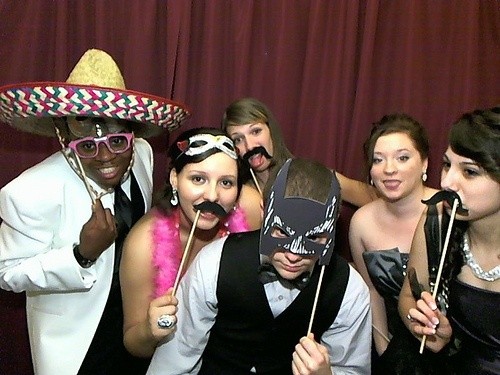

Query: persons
346 113 445 353
1 106 156 375
384 106 499 375
118 125 263 375
145 156 373 375
217 97 379 209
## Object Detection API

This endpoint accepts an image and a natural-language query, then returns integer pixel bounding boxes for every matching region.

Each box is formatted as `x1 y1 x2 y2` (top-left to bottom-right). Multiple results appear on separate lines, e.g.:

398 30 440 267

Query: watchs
73 245 97 268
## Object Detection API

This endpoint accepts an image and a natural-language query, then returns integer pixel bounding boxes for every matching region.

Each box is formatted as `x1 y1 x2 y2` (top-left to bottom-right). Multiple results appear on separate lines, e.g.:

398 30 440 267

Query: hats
1 49 192 138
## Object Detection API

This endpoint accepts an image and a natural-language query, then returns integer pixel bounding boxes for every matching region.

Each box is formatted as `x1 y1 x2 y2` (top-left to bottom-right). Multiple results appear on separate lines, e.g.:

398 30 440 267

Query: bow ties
257 263 311 291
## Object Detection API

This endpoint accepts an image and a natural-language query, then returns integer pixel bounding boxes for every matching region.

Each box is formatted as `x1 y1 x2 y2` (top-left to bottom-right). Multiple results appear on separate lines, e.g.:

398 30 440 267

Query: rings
406 313 413 320
158 315 174 328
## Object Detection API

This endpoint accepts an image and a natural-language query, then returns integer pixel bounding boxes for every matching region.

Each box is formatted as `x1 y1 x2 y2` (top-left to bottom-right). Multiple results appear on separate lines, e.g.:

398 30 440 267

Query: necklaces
461 232 499 282
